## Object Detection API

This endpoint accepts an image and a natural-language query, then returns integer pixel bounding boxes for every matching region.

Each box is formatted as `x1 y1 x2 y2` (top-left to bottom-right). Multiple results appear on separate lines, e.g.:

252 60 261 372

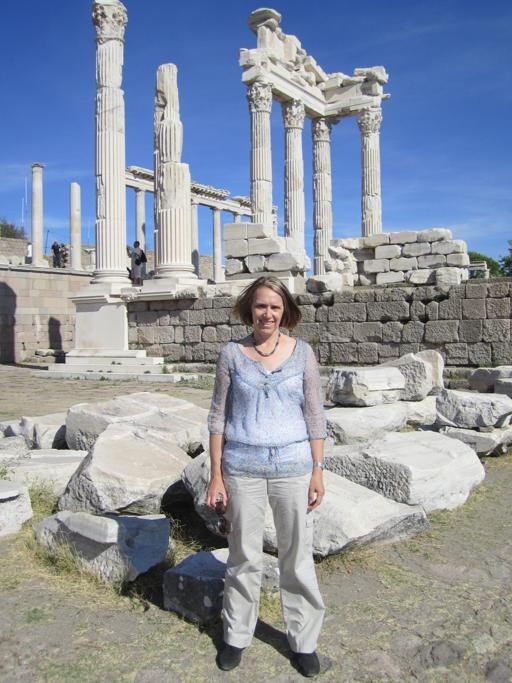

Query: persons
58 243 68 268
25 241 32 264
127 240 146 286
202 276 327 679
51 240 61 268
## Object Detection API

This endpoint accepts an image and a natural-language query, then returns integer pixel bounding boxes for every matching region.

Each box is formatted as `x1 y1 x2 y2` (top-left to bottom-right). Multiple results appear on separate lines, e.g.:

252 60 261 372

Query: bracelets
313 461 325 470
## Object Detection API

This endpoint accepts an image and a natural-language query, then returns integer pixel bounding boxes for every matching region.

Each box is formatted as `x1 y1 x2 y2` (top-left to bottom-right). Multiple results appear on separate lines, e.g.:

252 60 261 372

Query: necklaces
250 329 282 357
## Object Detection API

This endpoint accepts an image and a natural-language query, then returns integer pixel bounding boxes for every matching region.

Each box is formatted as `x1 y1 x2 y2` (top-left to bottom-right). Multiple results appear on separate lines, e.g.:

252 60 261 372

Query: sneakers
217 643 242 671
294 652 321 677
